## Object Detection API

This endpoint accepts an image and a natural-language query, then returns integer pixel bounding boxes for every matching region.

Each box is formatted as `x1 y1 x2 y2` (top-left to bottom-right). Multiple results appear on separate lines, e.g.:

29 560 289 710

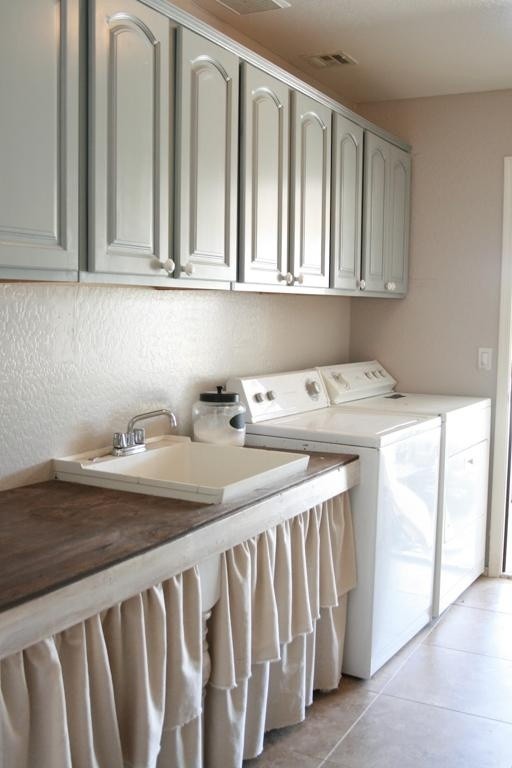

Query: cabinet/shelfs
0 0 78 283
362 117 411 297
238 42 331 295
331 98 365 297
79 0 237 291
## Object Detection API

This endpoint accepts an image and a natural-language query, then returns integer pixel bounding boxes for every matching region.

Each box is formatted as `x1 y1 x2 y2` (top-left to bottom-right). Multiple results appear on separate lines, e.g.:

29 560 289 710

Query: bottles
194 385 247 446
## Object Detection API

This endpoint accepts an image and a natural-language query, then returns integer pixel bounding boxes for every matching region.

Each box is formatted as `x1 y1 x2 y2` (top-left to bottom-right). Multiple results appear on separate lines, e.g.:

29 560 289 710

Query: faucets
113 409 177 454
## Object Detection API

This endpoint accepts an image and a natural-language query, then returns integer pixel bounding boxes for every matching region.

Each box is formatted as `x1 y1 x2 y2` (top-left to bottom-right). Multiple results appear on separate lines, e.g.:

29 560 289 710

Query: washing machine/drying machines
317 359 491 618
226 367 441 680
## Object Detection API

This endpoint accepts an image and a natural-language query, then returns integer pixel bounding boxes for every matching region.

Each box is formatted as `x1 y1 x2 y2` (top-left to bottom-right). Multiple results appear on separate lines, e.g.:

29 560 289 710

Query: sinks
52 435 310 508
